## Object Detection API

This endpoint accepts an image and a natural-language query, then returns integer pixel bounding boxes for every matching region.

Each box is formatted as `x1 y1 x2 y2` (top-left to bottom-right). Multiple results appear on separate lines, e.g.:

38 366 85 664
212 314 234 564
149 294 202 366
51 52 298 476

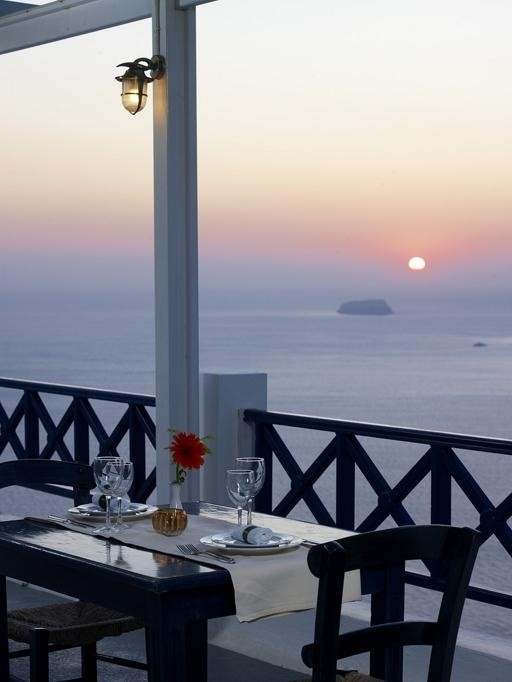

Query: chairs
296 516 485 682
1 458 157 681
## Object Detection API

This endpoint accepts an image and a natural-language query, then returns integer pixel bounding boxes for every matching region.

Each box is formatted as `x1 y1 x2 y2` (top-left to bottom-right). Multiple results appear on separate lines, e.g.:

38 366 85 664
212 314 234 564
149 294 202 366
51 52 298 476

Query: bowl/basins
152 508 188 537
153 550 180 566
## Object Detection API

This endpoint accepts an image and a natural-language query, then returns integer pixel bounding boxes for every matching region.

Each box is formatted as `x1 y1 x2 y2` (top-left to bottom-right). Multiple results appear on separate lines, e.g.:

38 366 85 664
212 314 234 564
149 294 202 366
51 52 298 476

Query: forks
175 543 237 565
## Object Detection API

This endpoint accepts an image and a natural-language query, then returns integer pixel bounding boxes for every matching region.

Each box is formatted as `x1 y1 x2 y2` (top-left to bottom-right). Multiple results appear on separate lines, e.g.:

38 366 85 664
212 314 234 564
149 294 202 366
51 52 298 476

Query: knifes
48 512 97 531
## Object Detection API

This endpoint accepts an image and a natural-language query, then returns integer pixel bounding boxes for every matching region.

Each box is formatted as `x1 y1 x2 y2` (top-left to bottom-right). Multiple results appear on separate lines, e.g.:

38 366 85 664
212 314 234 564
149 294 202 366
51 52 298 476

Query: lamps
115 53 167 118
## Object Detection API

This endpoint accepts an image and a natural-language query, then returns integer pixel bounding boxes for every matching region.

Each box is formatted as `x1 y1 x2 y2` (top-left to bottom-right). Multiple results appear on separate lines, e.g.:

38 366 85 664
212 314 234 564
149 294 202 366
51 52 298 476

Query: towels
91 494 130 512
227 523 274 546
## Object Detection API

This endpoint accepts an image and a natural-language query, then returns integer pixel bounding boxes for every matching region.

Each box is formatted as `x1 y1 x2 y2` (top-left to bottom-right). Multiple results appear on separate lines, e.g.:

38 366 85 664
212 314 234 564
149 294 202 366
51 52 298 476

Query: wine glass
102 539 132 570
224 455 265 525
91 457 136 533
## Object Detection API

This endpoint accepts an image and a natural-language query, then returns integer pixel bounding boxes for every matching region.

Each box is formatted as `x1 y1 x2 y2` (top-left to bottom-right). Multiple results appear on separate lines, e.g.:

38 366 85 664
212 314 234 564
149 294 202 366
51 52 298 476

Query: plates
200 532 305 555
69 501 157 522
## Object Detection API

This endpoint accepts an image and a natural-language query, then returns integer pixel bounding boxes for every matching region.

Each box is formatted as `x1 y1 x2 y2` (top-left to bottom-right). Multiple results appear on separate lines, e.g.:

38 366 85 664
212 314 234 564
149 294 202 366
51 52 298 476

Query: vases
168 482 184 513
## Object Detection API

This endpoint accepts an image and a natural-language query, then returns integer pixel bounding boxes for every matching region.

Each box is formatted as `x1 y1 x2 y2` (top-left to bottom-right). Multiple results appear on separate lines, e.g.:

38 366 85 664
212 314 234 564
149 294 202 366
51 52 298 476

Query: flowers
161 423 216 485
